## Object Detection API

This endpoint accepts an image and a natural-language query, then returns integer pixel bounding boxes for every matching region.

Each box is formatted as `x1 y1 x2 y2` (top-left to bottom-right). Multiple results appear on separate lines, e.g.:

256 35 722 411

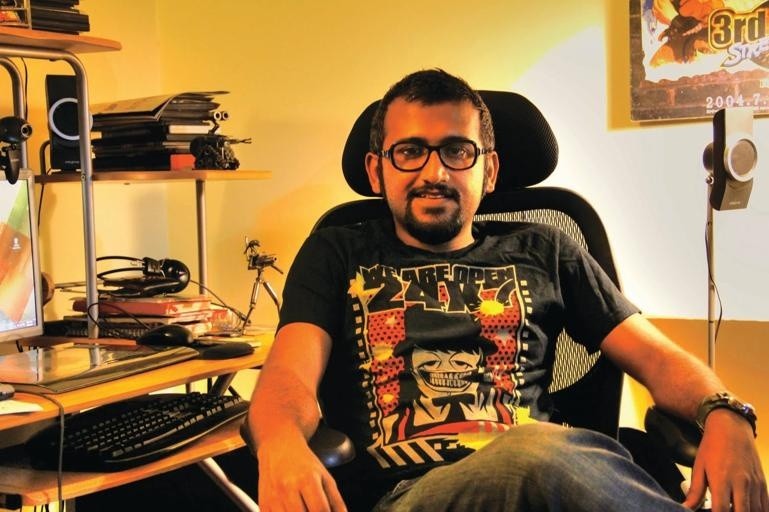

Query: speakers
45 75 81 175
703 107 758 211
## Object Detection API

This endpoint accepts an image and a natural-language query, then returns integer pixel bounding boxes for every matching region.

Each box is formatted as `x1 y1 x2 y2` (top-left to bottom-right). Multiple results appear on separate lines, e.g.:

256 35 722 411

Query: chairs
239 90 712 511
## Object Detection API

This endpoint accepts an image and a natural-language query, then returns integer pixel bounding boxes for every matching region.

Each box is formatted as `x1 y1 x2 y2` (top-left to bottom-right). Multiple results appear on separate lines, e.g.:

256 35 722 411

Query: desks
0 26 276 512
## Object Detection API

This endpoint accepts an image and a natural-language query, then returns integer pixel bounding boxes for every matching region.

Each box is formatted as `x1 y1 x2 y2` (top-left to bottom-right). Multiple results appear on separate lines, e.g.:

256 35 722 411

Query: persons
649 0 725 66
246 66 769 511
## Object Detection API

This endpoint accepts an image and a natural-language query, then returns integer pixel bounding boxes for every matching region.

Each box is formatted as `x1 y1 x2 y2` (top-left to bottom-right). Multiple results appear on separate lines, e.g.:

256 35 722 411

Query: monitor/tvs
1 170 44 344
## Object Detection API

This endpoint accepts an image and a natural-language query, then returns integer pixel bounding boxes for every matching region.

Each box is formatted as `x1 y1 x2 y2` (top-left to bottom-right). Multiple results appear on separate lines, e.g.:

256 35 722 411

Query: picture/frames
603 1 769 130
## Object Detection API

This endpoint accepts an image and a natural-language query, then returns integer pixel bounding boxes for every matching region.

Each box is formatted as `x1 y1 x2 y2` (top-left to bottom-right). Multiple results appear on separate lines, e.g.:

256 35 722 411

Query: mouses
137 325 193 346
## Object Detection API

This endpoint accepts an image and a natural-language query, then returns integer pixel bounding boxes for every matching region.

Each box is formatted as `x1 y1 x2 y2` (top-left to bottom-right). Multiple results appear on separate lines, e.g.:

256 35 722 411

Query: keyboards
30 392 250 471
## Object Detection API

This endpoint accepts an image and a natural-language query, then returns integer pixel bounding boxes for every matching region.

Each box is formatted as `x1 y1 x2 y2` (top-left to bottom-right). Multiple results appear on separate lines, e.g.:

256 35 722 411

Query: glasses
375 140 488 171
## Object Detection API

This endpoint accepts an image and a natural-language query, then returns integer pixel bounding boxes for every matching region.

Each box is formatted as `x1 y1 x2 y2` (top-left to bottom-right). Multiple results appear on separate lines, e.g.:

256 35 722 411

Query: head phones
97 255 190 298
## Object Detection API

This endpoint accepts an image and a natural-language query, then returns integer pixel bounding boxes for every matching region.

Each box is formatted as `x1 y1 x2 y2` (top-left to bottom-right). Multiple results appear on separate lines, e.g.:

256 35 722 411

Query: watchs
695 391 757 439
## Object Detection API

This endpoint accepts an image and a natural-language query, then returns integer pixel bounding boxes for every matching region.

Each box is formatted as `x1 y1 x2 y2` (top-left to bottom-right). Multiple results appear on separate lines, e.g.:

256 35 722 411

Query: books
62 294 217 342
88 91 233 171
